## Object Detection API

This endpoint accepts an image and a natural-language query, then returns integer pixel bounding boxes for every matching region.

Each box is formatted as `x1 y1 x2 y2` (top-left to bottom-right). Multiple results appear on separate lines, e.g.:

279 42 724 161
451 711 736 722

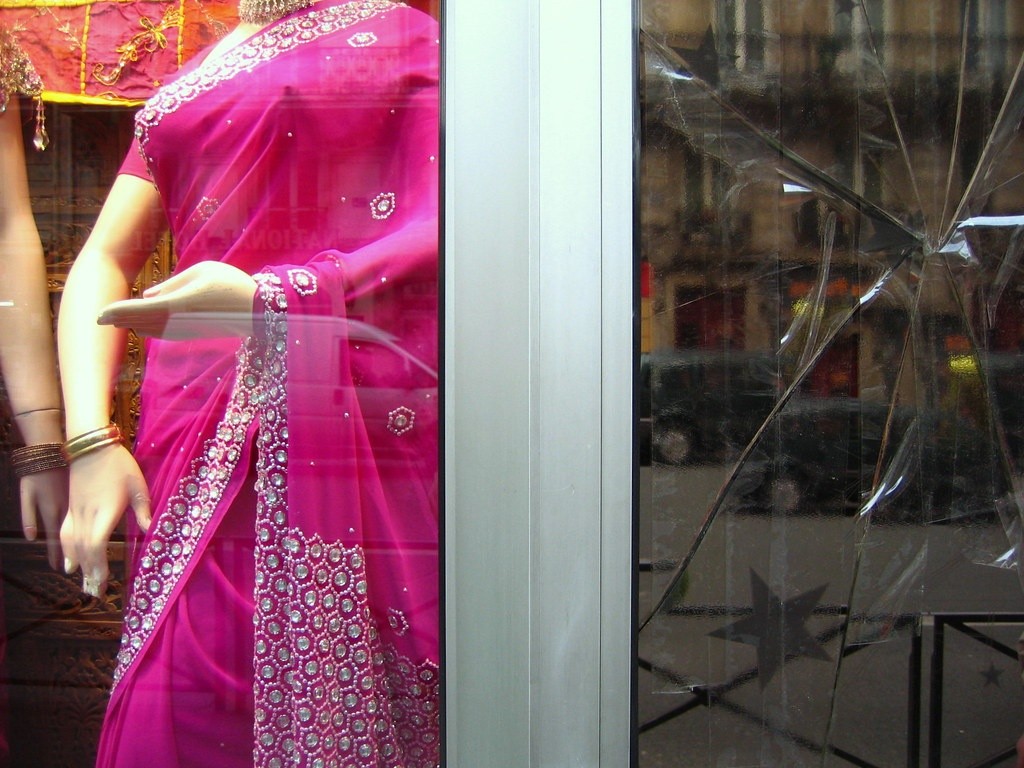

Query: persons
57 0 445 767
0 21 63 570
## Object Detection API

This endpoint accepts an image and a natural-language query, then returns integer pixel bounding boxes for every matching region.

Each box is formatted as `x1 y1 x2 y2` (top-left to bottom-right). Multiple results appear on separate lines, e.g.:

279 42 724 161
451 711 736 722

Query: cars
742 400 1004 523
640 349 778 468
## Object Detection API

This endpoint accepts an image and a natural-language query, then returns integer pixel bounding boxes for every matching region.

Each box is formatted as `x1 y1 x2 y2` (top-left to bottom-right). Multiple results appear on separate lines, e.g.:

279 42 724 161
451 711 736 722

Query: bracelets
10 443 66 475
60 422 123 463
